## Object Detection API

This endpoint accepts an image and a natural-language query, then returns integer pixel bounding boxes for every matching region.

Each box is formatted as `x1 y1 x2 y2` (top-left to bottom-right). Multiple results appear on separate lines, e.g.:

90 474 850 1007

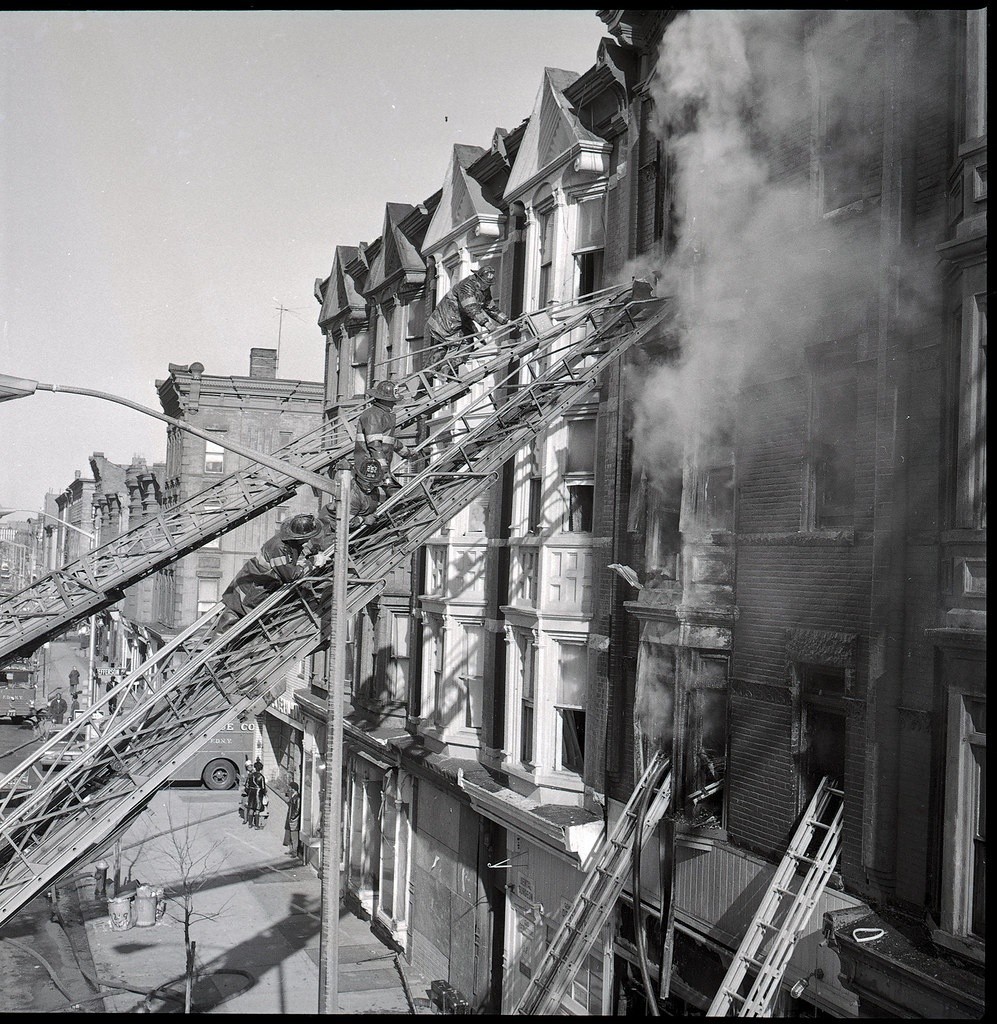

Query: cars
41 723 93 769
1 560 14 601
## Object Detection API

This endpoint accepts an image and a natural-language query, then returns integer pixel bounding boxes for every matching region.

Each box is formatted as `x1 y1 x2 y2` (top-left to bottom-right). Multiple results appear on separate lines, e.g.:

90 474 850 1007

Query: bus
0 656 40 725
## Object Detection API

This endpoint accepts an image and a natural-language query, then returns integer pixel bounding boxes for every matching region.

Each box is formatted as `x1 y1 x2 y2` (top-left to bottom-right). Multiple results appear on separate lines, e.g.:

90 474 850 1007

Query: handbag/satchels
263 794 269 806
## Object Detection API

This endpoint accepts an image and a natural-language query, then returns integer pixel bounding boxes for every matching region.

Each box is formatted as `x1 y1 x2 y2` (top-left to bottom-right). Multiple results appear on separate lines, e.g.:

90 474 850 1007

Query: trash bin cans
134 896 155 925
108 899 133 932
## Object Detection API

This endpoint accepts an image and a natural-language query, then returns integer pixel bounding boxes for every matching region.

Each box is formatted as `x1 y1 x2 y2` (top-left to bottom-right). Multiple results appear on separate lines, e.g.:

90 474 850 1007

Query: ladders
706 773 846 1016
513 751 671 1014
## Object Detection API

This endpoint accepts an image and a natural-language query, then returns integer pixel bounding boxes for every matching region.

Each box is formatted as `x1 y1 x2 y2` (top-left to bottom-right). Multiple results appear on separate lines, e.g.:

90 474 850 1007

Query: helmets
470 265 497 286
366 381 404 402
354 458 383 484
281 514 321 540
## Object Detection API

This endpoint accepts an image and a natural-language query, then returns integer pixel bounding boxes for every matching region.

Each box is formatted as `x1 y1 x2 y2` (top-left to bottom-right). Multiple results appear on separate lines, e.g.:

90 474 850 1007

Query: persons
70 694 79 720
354 379 421 500
68 666 80 695
106 675 120 714
239 760 254 825
245 762 267 830
425 264 521 381
311 459 384 550
211 511 321 649
50 693 67 723
284 782 301 855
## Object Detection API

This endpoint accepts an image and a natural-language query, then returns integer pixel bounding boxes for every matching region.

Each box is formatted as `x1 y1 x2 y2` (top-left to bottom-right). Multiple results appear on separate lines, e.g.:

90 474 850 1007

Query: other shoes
248 824 253 828
284 851 296 858
255 826 262 830
242 821 247 824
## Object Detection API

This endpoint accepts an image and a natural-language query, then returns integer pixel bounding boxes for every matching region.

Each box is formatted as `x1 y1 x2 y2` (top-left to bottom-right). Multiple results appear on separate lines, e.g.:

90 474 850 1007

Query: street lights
43 642 49 700
1 373 355 1015
0 503 99 763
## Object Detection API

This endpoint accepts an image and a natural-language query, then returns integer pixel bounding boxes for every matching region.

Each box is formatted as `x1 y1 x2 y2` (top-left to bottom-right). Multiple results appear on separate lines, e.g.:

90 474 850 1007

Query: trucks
97 712 256 793
